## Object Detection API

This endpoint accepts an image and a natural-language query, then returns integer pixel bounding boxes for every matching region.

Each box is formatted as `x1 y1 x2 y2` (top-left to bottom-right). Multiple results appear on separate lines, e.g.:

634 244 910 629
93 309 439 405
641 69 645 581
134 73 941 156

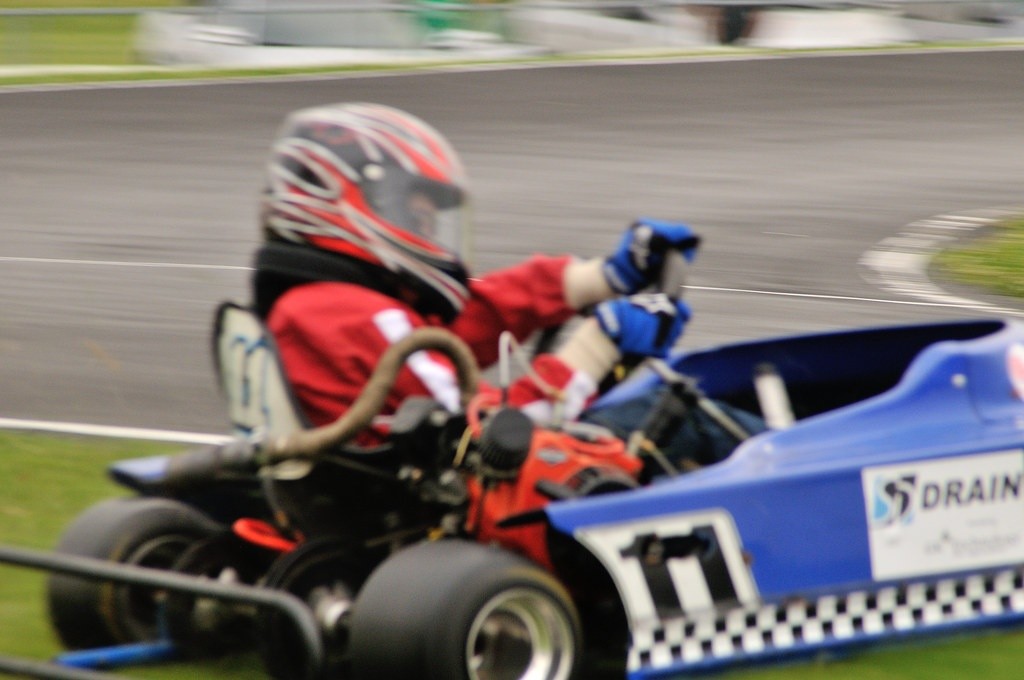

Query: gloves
591 293 690 357
603 219 696 295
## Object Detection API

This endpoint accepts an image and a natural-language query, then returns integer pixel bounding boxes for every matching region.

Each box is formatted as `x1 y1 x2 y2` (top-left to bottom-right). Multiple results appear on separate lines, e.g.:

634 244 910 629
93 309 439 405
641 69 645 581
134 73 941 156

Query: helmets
254 100 467 324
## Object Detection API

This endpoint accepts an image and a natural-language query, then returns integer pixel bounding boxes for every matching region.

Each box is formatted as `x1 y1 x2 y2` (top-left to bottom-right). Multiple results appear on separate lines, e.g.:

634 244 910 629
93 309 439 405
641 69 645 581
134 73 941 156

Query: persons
250 102 768 471
